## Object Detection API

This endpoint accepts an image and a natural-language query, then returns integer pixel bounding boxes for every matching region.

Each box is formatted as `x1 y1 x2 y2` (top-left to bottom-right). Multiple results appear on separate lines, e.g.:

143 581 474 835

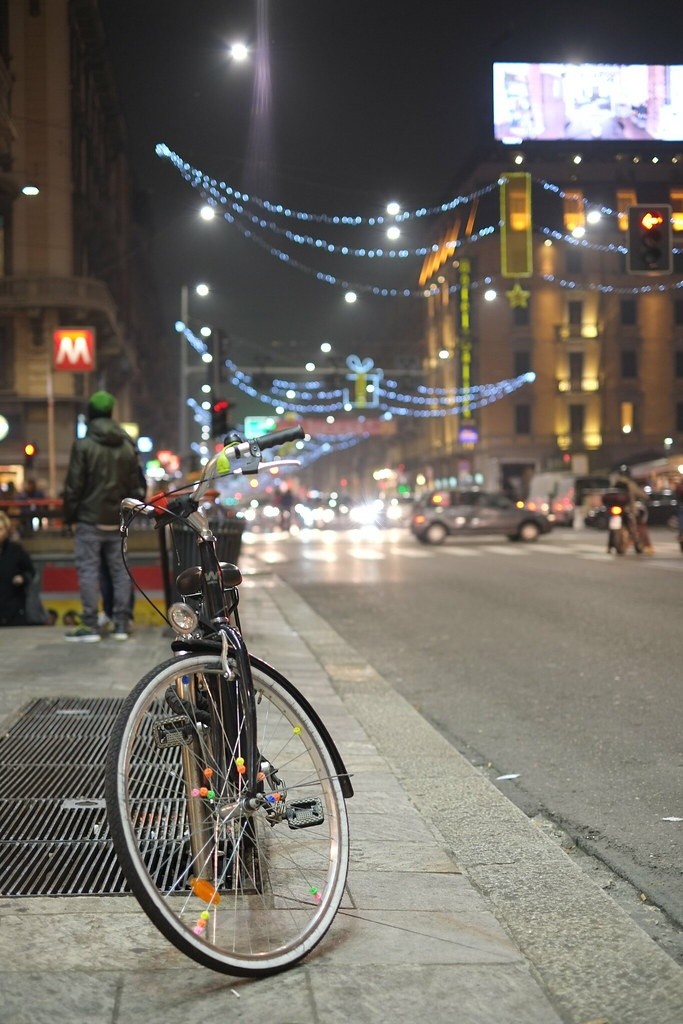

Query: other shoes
96 610 111 627
63 626 102 643
104 631 128 641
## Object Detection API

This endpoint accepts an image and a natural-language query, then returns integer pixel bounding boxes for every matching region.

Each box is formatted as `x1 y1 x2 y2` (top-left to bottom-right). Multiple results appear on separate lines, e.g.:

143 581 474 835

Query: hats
89 390 114 414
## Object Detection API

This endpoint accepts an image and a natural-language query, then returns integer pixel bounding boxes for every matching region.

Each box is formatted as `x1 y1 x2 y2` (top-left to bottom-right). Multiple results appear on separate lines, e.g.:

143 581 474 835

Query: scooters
601 486 640 554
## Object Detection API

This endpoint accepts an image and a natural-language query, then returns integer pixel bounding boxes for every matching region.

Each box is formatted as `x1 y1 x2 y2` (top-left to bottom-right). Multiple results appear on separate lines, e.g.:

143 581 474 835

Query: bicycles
105 426 357 980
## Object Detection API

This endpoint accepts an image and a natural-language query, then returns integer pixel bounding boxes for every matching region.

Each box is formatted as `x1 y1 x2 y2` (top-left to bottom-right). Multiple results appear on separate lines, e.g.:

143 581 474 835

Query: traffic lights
210 396 231 436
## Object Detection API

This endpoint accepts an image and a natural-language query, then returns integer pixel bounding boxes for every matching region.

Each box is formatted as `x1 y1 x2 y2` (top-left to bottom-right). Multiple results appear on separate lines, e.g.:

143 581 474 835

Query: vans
529 472 653 526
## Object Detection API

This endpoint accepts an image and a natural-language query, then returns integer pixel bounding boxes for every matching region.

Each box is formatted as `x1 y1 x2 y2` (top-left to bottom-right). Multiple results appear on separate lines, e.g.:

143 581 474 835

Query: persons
0 510 37 627
0 480 48 537
606 467 654 555
58 390 147 642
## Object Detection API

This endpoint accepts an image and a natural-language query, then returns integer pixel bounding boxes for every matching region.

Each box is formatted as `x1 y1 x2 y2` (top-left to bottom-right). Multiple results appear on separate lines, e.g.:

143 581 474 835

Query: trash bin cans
172 517 246 620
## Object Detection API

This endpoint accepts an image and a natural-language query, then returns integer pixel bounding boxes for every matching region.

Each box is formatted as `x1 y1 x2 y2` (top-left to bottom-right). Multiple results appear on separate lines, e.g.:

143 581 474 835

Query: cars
407 488 553 546
584 487 677 532
